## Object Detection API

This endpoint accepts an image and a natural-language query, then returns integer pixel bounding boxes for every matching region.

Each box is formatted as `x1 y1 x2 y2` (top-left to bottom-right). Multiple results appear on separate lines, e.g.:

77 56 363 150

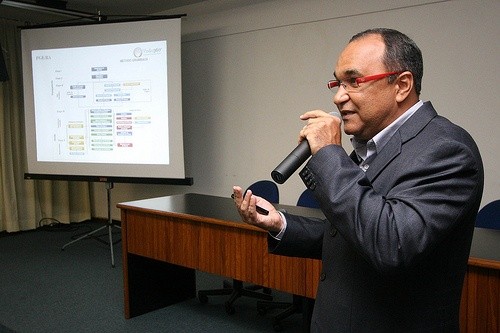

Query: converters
54 223 72 232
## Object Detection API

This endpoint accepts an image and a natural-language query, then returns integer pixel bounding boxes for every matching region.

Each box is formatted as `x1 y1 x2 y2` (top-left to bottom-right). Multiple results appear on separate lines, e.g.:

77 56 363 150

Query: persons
232 27 484 333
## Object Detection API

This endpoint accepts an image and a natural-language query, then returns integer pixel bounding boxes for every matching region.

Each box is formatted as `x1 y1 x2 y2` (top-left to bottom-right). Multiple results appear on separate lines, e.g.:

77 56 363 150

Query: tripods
61 181 122 267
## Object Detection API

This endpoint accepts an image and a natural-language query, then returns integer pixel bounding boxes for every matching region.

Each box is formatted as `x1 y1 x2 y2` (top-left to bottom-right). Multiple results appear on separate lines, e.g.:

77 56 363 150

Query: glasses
327 70 409 92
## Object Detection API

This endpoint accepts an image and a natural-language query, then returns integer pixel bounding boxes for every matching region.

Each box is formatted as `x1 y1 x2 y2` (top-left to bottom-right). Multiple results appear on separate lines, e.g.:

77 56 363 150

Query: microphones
270 111 343 184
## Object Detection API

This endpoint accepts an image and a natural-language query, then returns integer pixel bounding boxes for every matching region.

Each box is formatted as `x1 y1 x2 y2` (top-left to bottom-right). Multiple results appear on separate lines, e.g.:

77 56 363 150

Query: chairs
475 200 500 230
256 189 321 332
198 181 278 316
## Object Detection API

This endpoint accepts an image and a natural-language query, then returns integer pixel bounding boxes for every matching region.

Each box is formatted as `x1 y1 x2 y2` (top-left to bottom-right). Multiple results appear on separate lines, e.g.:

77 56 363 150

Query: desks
116 193 500 333
